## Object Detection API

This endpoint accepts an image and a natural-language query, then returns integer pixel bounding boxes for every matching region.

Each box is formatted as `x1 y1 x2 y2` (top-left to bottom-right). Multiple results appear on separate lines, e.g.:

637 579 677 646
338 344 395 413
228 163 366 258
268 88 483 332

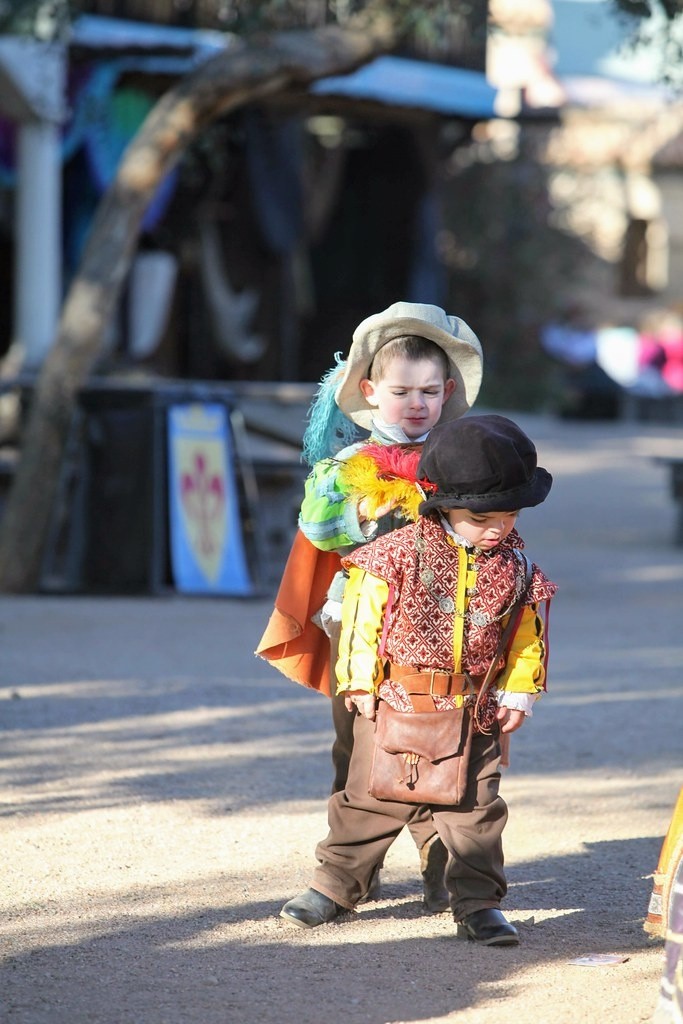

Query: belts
387 663 494 713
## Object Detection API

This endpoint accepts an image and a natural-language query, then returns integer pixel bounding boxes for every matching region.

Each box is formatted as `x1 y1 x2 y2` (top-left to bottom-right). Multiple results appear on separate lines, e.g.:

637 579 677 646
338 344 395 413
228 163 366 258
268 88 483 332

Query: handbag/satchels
367 551 532 804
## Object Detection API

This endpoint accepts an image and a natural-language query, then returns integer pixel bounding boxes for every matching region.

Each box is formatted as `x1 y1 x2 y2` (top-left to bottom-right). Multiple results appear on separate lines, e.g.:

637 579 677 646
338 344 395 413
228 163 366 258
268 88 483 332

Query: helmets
338 415 553 523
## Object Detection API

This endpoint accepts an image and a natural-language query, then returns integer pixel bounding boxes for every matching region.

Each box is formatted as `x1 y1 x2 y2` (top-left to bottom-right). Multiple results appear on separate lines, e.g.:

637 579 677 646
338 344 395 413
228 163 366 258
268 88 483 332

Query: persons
253 300 484 914
276 415 559 946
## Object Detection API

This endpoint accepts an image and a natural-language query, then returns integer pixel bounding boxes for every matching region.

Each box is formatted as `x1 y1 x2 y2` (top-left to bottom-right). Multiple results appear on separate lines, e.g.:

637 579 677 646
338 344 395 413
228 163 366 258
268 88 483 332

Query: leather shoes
456 908 521 947
422 880 453 912
357 873 379 904
278 887 346 928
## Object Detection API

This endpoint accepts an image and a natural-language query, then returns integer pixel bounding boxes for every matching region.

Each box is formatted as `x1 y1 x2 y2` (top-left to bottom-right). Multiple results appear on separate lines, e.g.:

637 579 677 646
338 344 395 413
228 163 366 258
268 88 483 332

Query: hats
300 301 484 466
